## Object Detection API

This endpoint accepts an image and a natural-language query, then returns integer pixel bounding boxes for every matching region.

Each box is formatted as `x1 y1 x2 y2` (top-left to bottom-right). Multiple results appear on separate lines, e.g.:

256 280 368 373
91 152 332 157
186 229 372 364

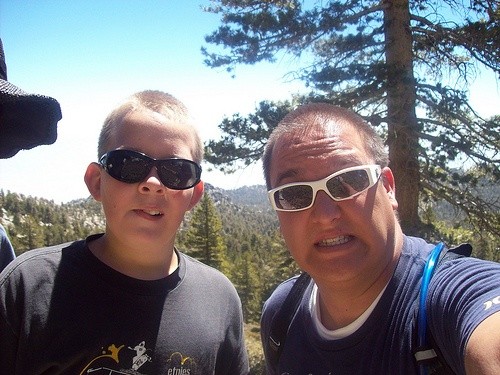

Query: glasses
267 164 382 212
99 149 202 190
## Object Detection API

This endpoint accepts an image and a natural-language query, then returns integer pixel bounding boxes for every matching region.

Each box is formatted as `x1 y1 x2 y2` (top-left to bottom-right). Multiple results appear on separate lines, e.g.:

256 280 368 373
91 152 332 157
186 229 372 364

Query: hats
1 38 61 159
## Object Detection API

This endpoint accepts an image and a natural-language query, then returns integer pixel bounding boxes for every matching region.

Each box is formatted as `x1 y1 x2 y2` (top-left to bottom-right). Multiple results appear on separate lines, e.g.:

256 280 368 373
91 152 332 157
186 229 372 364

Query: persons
258 101 500 374
0 89 251 375
